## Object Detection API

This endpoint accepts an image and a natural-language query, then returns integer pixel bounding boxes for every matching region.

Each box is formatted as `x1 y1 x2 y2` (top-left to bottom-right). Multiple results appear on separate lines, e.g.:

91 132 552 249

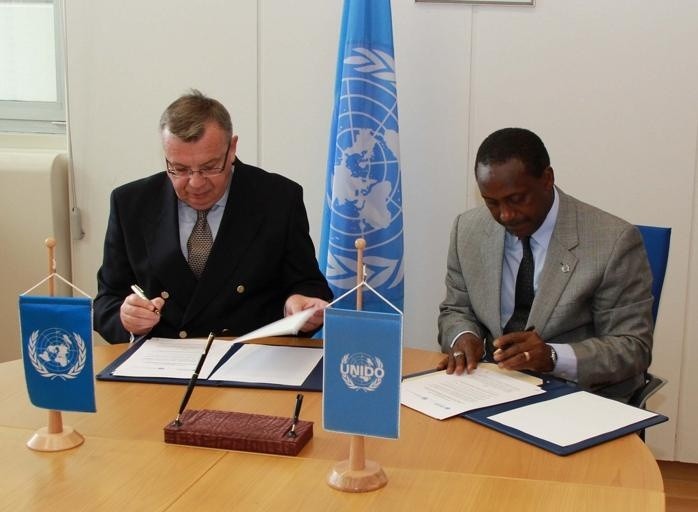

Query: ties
503 235 533 336
187 208 214 281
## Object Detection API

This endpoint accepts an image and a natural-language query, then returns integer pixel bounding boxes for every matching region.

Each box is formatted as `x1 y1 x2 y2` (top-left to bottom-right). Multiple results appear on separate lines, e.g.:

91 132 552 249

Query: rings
452 350 465 358
522 352 530 362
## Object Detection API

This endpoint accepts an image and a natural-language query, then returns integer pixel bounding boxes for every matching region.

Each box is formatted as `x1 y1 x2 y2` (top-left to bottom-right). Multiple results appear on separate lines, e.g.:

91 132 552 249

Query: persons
91 85 334 345
436 127 654 404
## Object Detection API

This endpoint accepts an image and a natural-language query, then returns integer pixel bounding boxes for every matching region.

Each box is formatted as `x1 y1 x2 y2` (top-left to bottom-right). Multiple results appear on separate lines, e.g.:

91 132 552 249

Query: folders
96 334 324 393
401 359 669 456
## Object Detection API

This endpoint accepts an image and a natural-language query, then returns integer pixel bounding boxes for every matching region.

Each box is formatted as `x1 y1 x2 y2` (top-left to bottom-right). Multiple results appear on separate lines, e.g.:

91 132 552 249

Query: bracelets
547 346 556 370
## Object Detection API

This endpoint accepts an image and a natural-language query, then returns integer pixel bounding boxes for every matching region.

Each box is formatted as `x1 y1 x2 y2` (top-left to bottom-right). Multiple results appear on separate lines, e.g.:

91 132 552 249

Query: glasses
166 142 230 177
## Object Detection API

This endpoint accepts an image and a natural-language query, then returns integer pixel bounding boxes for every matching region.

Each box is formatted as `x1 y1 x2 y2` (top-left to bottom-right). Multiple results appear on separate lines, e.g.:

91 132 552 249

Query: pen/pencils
492 326 535 356
175 333 215 423
131 284 160 315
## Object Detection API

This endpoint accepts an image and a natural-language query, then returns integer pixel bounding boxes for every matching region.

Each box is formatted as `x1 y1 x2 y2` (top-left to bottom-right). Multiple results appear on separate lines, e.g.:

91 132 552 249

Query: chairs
626 223 672 445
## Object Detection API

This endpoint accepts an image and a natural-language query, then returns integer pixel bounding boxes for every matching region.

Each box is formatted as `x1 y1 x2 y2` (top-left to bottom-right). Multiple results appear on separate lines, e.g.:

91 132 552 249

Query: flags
309 0 403 339
19 295 95 412
323 308 400 442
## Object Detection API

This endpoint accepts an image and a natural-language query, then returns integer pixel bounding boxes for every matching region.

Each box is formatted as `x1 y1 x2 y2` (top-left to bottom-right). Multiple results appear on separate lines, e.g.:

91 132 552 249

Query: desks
0 336 667 512
